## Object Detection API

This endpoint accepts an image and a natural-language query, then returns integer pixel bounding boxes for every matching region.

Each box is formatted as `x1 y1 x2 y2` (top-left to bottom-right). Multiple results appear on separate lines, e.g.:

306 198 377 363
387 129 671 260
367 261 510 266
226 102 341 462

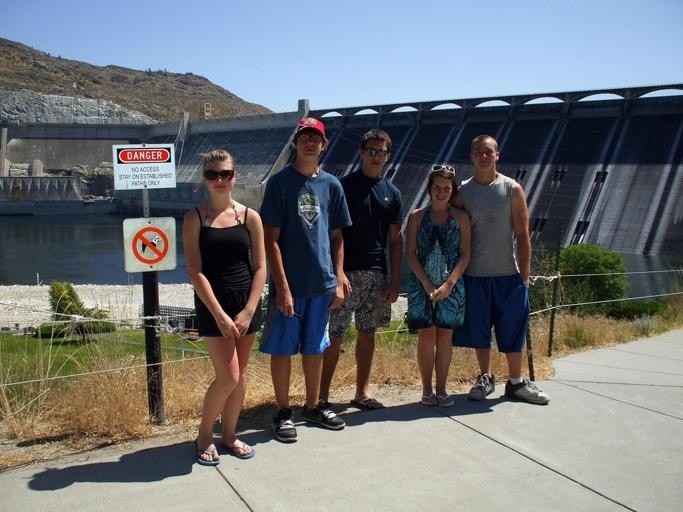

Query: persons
450 133 552 406
316 128 406 411
181 148 268 466
404 163 472 409
255 116 353 444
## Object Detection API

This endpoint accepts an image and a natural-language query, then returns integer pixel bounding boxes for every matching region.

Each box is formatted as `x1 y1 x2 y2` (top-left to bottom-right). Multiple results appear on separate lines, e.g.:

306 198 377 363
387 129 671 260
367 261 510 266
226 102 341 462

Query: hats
297 116 326 139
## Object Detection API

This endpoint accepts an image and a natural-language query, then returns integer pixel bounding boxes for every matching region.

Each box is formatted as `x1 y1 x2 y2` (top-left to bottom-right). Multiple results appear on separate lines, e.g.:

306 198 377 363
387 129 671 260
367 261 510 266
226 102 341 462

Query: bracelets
445 278 453 291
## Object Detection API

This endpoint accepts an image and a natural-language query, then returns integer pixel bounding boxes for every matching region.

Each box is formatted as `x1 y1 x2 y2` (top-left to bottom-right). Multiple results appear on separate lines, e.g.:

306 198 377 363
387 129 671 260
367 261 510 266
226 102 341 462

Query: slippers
349 396 385 410
221 435 256 459
195 437 220 466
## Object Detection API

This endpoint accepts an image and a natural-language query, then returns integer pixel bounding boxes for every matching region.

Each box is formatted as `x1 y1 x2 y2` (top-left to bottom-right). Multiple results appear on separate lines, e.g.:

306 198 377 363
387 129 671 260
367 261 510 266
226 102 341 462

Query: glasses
430 163 457 175
204 169 235 180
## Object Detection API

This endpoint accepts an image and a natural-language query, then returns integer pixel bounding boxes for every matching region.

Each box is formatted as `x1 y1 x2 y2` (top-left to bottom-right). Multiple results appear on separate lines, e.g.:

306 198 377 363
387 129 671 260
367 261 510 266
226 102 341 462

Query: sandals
436 393 454 407
421 393 438 406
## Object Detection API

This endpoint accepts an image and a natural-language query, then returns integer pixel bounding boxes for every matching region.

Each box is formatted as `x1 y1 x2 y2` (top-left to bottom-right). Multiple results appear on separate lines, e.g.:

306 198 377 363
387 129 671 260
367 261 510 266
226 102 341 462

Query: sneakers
468 372 496 401
271 408 298 442
303 404 346 429
503 379 551 405
